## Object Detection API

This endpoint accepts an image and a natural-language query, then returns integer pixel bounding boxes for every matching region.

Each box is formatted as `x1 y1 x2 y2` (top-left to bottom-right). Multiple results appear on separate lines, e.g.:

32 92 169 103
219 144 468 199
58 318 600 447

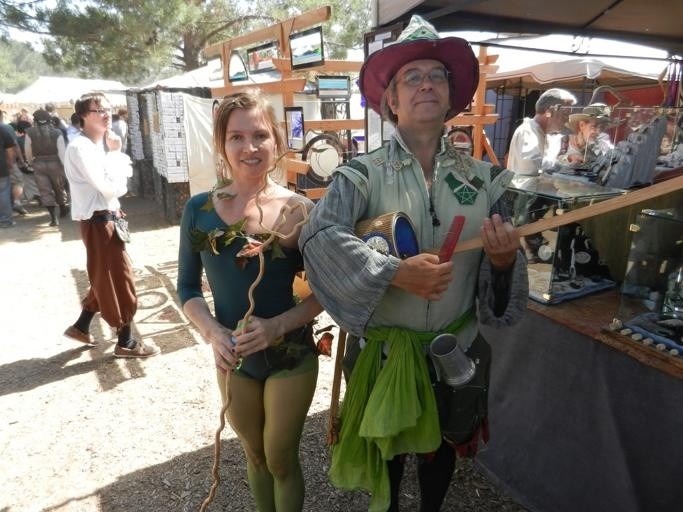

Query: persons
62 93 162 359
1 100 137 228
175 89 324 512
557 100 629 167
297 14 528 512
505 88 583 263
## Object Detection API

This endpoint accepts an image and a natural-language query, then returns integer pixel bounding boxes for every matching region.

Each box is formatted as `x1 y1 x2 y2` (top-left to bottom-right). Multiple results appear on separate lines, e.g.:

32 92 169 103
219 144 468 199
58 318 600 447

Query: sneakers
65 325 101 347
12 202 27 214
113 340 162 358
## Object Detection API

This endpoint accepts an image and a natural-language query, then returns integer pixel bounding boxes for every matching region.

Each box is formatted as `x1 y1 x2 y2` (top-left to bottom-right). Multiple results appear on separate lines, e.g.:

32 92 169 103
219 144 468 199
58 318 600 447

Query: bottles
227 321 247 371
427 333 476 388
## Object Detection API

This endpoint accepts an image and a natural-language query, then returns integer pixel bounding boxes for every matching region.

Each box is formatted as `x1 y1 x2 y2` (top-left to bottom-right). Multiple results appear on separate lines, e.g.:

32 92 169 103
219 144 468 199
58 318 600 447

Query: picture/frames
316 74 351 99
288 25 325 71
246 39 279 74
283 107 306 150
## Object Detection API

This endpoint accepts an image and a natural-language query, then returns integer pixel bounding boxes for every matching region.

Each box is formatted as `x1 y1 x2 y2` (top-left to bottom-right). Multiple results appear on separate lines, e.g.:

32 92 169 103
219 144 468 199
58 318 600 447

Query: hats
360 14 479 124
564 104 627 133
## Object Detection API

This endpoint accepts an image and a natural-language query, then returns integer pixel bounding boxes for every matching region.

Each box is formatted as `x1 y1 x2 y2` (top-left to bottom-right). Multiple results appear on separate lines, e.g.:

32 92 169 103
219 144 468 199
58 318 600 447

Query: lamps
229 50 248 82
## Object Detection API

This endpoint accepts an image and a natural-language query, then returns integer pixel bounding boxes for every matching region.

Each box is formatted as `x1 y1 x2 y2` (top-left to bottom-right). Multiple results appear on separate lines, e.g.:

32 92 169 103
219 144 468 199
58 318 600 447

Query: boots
48 204 68 226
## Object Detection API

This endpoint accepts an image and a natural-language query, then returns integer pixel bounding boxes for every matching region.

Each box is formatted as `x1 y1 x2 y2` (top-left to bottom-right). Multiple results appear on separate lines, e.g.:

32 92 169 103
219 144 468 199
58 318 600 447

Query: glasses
394 68 453 89
88 107 115 117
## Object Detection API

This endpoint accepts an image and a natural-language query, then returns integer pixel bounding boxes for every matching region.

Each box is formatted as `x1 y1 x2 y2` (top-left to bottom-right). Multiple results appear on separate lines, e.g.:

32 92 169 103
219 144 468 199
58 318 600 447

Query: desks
448 255 682 512
